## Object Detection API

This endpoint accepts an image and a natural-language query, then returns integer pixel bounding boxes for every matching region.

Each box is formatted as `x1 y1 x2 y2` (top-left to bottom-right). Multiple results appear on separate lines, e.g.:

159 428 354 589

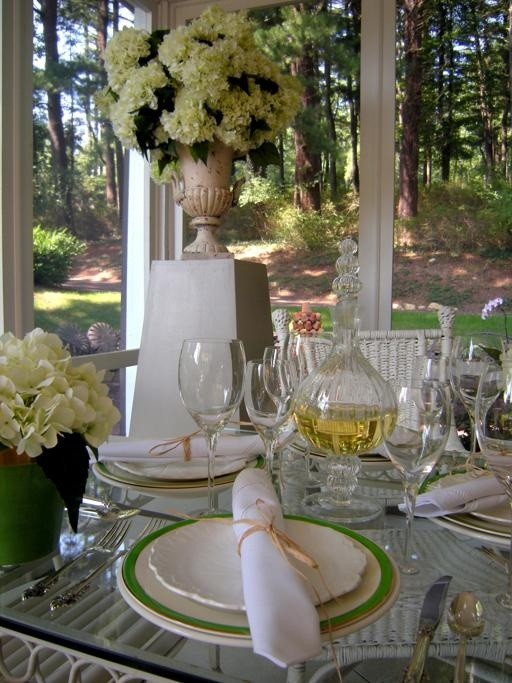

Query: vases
163 137 238 254
1 448 67 572
499 348 512 388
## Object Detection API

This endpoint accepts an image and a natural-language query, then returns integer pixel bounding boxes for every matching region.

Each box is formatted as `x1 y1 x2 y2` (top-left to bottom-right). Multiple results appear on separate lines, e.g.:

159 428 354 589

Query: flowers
0 326 121 457
482 299 511 356
97 6 308 183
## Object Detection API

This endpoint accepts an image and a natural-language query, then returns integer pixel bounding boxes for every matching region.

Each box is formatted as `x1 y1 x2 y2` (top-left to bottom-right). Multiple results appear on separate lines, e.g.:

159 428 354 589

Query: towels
96 435 266 461
397 476 508 517
231 468 323 666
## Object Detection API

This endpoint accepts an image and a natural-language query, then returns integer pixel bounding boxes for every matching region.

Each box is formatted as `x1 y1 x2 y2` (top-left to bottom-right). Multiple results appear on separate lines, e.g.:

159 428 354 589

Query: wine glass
384 381 451 578
476 358 512 611
178 338 248 517
447 339 506 453
265 347 302 418
283 332 328 490
244 361 296 485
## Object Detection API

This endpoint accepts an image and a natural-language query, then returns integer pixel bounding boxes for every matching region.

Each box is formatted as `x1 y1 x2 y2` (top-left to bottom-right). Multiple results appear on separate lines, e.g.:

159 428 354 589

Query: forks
23 520 133 601
50 519 168 612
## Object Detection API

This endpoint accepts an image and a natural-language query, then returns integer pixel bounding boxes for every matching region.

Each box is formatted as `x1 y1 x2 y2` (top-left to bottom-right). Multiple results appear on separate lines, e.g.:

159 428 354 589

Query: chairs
271 307 511 681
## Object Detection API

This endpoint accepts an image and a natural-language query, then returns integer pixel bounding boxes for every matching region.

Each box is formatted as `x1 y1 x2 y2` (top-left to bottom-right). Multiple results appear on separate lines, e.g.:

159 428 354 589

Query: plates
119 512 401 650
92 454 264 500
418 467 511 548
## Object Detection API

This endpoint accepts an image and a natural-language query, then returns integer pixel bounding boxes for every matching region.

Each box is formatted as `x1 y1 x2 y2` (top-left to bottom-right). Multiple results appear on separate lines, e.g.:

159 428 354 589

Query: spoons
447 591 486 683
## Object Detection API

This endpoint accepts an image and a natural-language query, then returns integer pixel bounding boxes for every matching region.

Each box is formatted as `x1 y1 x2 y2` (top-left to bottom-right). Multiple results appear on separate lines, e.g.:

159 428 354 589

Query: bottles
291 233 399 524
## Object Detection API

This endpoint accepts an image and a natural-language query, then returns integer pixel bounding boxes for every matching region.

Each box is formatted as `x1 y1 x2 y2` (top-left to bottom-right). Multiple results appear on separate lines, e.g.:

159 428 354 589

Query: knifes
404 576 454 683
